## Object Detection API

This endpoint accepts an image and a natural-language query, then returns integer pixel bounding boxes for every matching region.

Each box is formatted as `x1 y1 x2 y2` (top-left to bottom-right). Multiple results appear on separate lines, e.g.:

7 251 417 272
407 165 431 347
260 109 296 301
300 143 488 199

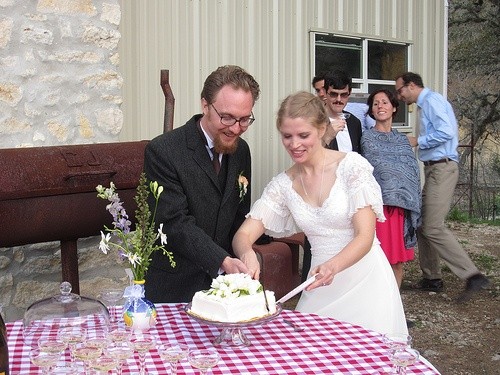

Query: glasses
326 90 350 98
396 82 409 94
211 103 255 127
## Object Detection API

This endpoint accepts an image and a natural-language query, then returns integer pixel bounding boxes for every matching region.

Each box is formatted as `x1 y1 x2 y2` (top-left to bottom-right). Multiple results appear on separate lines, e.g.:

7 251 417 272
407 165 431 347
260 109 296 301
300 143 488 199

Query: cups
338 113 350 120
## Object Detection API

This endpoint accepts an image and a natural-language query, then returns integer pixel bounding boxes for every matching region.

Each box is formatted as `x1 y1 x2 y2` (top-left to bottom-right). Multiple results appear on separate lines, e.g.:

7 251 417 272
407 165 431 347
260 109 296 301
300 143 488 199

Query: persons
144 64 261 303
395 72 489 306
360 90 422 329
321 70 362 153
233 91 409 344
312 75 376 131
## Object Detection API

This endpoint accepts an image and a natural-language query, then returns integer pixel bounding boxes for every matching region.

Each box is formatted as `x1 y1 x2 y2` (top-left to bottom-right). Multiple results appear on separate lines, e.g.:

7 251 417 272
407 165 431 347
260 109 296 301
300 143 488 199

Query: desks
6 303 442 375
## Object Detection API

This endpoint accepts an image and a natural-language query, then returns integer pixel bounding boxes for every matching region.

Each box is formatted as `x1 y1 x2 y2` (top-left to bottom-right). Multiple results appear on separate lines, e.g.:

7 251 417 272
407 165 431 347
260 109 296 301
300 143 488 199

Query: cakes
190 273 276 322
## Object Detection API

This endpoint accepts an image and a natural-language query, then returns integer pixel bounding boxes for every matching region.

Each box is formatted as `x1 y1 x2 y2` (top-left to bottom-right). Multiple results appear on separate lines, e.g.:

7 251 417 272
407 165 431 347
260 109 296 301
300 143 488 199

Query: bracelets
322 135 329 146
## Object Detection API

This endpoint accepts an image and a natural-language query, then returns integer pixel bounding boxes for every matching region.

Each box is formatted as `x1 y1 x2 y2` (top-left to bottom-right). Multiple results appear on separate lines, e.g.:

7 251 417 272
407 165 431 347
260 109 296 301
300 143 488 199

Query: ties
210 147 220 177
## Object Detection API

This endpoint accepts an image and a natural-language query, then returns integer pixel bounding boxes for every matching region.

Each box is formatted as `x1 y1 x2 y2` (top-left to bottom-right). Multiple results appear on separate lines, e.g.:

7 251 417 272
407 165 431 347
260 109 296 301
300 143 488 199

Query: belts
424 158 452 165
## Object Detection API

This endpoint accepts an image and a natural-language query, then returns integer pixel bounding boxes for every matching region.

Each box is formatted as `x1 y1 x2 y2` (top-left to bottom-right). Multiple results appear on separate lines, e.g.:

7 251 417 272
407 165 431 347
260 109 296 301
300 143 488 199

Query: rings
322 282 326 286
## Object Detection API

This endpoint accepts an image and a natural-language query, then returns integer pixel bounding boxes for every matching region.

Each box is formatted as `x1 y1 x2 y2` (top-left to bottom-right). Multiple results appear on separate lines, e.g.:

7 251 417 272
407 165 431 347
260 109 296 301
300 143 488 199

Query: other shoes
458 275 489 305
404 278 443 292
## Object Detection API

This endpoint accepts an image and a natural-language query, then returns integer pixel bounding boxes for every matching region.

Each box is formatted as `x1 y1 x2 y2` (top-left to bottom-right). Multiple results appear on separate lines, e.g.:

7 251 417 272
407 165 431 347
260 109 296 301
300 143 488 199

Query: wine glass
158 342 189 375
188 349 222 375
28 326 158 375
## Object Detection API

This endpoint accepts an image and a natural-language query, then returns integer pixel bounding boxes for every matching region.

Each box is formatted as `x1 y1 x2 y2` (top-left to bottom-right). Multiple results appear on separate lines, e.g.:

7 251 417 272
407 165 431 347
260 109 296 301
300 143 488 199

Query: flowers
238 171 248 203
96 173 176 282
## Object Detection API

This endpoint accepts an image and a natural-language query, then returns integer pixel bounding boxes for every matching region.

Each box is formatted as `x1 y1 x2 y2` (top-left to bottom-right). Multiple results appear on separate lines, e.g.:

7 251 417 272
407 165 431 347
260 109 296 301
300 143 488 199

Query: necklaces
299 149 326 206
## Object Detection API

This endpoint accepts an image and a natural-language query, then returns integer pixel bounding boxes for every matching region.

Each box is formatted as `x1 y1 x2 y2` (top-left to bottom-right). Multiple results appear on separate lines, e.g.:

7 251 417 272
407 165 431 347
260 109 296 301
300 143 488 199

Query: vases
125 280 157 329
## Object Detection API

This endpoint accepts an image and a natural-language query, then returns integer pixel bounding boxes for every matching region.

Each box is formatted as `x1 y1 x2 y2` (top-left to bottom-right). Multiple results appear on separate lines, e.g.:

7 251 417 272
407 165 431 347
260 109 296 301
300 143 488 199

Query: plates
276 273 319 305
